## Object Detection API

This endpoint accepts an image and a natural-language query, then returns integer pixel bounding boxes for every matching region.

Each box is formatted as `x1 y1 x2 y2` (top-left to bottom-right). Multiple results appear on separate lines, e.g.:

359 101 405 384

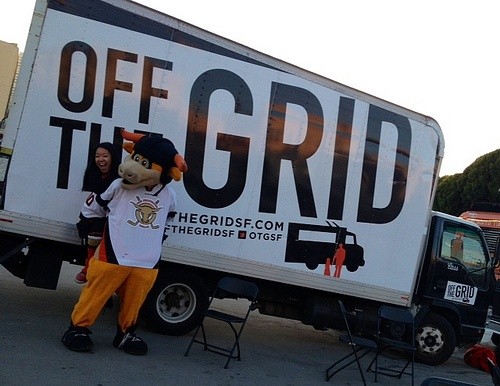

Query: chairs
366 305 417 386
326 300 377 386
183 276 259 369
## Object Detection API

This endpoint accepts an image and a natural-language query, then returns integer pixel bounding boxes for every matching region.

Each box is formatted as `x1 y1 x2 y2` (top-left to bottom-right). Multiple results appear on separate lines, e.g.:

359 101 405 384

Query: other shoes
113 322 149 355
62 321 95 351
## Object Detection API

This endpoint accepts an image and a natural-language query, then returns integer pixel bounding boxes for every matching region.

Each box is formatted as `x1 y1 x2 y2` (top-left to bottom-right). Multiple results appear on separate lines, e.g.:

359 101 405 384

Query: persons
74 142 120 284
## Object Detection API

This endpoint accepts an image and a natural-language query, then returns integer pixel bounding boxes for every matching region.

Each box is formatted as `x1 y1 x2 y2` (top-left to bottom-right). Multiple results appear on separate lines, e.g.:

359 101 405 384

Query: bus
452 210 500 315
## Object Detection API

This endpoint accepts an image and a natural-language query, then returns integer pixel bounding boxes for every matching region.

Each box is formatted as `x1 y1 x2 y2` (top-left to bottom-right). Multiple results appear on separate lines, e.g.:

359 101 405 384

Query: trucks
0 1 500 368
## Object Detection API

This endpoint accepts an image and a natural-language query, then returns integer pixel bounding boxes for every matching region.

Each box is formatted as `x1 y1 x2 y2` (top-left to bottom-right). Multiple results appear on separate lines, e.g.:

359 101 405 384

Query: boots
74 234 103 284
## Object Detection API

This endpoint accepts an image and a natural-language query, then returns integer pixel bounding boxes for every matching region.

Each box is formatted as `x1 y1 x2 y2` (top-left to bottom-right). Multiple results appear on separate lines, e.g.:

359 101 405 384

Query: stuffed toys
61 128 188 355
